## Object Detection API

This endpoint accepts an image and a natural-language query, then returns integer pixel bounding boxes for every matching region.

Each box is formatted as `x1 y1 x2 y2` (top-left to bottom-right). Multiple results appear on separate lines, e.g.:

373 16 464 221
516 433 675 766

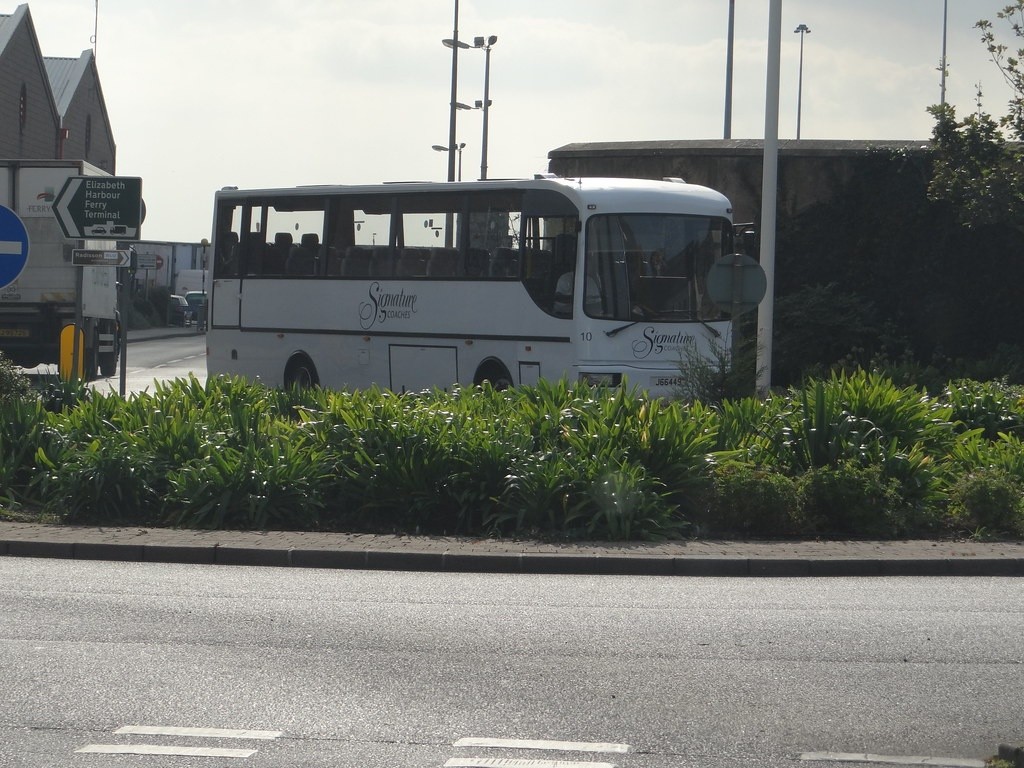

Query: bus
205 172 731 404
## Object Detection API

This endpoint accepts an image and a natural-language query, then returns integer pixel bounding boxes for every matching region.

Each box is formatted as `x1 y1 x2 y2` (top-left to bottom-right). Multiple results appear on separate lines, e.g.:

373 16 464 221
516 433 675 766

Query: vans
171 294 192 319
185 291 207 319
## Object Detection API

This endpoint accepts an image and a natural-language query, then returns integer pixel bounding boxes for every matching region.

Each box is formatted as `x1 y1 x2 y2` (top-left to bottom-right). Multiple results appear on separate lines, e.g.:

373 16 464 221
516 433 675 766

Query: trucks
0 159 119 382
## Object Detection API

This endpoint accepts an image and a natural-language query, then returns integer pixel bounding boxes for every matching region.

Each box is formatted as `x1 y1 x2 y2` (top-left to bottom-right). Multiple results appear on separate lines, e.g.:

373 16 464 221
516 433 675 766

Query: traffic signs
52 174 142 242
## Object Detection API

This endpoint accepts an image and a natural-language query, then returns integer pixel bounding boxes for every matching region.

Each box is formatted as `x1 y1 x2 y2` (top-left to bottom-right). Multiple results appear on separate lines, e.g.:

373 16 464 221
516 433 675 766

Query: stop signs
156 255 163 269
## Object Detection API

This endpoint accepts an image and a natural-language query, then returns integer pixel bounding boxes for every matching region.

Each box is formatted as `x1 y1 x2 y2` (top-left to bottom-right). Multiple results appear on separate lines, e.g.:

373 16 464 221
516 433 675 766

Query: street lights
443 35 497 179
794 24 812 139
433 142 466 182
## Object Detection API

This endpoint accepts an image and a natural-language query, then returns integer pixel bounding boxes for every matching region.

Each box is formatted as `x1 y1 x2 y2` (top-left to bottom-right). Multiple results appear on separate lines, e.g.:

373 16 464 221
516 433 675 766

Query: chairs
228 228 587 308
